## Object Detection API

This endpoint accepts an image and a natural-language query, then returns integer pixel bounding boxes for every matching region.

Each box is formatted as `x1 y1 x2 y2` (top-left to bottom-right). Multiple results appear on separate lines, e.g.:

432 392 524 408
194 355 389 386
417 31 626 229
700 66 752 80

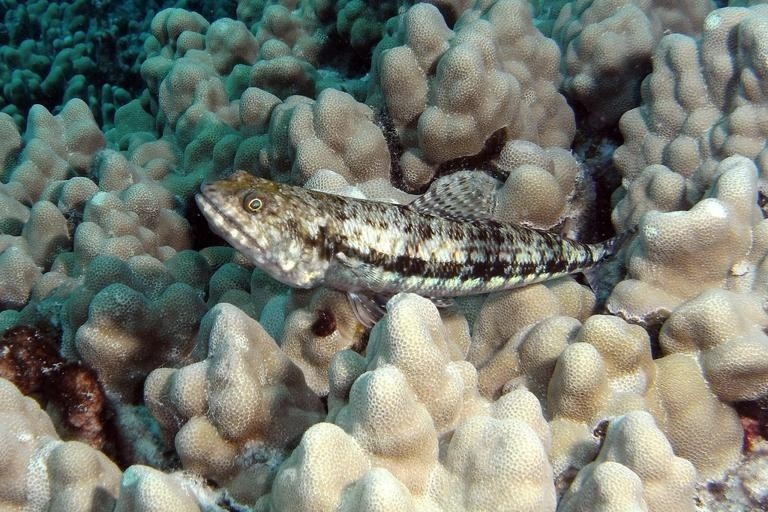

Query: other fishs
191 124 642 330
